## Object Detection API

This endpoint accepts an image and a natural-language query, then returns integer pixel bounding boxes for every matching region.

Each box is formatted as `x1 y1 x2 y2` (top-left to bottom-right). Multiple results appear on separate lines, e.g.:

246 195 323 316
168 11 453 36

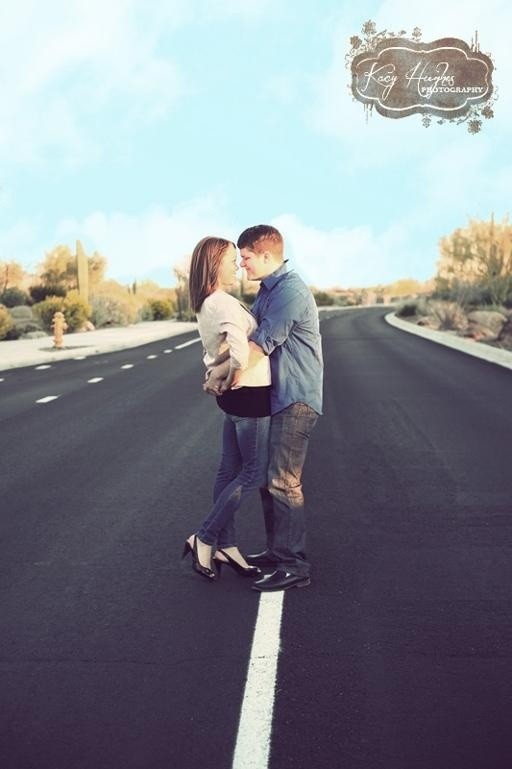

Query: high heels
181 533 215 581
213 548 261 577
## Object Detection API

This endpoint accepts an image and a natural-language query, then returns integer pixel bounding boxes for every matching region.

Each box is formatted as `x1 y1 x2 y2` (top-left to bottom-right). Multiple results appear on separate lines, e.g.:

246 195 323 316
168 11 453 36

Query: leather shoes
252 569 309 592
247 552 276 565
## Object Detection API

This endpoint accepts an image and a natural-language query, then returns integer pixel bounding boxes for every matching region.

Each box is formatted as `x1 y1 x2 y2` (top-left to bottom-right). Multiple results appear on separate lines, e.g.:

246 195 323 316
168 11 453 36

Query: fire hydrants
49 312 68 349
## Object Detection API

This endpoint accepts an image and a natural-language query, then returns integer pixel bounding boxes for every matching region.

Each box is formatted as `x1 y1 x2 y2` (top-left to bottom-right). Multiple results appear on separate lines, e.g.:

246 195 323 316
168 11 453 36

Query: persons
181 236 272 581
202 224 325 592
